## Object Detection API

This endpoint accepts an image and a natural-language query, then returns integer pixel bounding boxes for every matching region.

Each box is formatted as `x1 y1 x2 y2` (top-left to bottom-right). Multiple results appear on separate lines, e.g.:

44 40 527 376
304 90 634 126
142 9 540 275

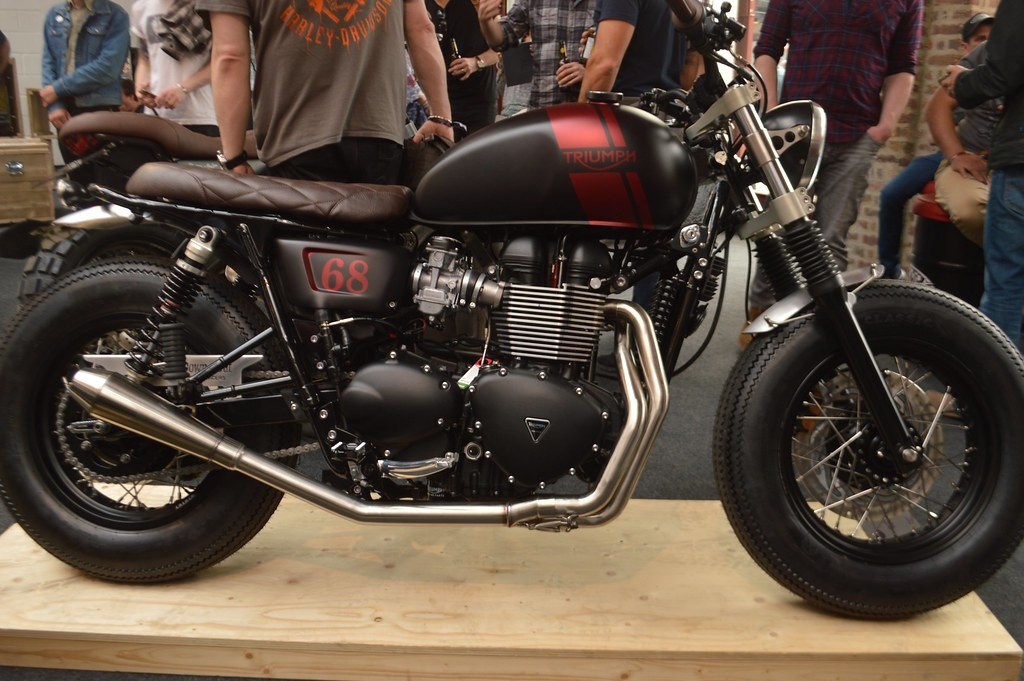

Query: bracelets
949 152 965 161
177 84 188 93
427 116 453 127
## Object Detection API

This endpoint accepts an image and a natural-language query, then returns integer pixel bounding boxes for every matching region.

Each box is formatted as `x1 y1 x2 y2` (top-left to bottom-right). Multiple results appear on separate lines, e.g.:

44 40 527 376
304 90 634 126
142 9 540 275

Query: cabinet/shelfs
0 88 57 225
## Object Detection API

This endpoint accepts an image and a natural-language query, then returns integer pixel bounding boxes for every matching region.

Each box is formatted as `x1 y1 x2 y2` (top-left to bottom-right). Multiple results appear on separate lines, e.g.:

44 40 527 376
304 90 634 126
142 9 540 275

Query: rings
571 74 574 78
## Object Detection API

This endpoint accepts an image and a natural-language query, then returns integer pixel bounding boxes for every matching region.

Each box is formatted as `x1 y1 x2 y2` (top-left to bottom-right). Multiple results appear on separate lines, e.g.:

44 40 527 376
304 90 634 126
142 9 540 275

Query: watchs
215 150 247 171
476 56 484 68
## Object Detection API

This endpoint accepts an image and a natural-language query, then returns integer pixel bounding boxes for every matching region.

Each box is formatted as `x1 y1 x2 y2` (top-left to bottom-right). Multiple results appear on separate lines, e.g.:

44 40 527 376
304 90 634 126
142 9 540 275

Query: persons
738 0 923 430
877 0 1024 418
405 0 705 138
39 0 129 165
194 0 456 189
128 0 220 136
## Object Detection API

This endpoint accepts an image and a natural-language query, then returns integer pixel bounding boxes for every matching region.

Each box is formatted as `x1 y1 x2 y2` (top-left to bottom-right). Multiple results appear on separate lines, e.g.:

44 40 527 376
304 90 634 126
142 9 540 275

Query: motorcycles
13 98 260 326
0 1 1024 623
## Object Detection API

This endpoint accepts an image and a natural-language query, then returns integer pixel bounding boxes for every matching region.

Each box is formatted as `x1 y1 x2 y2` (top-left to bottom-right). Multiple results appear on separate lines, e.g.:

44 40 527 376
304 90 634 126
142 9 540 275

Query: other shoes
793 386 824 442
897 385 960 417
740 306 771 352
898 267 906 281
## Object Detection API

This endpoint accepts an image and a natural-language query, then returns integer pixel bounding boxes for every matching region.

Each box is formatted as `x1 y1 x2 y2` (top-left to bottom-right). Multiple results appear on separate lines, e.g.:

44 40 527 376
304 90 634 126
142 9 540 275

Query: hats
961 12 996 44
